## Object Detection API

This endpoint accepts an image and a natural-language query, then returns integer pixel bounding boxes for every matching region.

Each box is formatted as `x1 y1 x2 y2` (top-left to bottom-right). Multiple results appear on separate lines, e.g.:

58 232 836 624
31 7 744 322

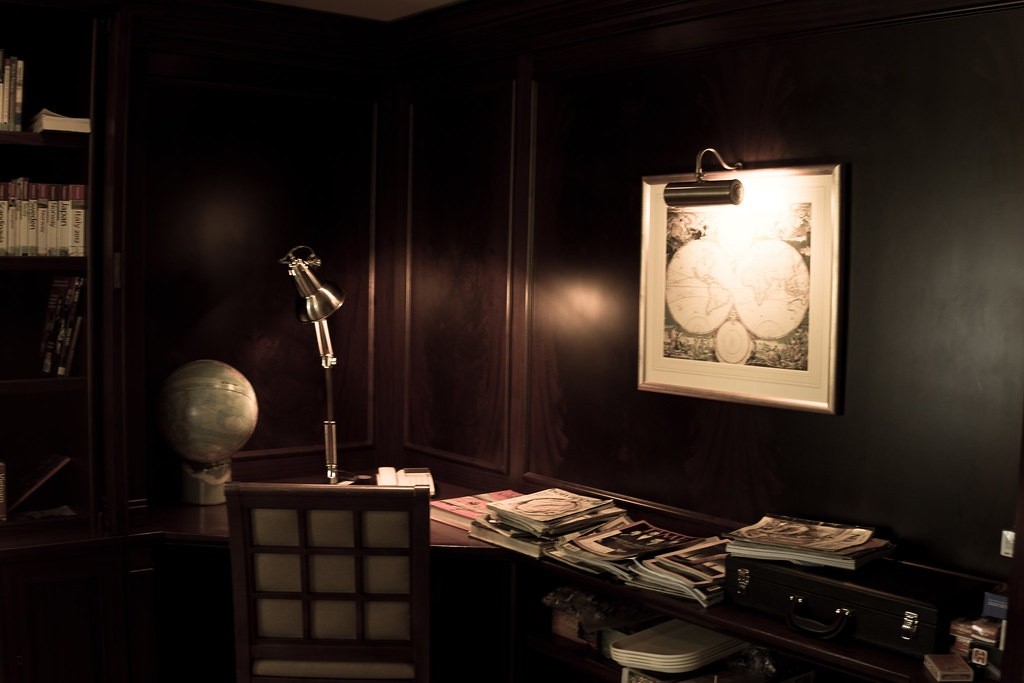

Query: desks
0 466 1024 683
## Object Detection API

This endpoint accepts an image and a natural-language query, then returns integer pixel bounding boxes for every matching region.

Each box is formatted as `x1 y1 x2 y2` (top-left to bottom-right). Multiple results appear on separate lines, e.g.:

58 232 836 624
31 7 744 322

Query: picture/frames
636 163 841 416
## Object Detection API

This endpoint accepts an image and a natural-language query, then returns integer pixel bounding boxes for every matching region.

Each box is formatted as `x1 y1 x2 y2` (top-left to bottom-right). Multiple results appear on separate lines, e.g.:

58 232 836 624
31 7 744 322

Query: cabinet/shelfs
0 545 129 683
0 0 100 534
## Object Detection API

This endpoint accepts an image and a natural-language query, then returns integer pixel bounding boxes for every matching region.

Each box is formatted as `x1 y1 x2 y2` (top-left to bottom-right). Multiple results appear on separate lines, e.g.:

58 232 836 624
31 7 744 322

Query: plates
609 618 748 673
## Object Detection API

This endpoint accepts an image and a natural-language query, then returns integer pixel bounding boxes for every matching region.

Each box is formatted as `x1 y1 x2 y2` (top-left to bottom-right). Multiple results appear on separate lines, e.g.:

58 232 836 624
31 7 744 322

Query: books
429 485 894 609
0 61 90 258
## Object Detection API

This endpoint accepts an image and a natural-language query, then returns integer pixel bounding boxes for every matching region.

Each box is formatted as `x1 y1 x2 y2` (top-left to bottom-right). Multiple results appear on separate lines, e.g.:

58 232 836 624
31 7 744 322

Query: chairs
223 480 431 683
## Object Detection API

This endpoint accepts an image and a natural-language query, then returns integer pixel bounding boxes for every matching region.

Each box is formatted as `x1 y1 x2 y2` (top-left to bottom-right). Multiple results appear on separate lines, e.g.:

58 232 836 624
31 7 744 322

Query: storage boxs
923 653 974 682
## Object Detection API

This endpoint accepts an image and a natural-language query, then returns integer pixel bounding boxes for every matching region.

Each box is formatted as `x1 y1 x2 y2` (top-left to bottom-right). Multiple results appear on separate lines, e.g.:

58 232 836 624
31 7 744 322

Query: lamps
663 147 745 208
278 245 348 484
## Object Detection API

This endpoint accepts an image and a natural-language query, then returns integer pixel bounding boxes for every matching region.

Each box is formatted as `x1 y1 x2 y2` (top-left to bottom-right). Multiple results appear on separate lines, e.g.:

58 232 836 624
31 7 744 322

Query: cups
356 475 373 485
